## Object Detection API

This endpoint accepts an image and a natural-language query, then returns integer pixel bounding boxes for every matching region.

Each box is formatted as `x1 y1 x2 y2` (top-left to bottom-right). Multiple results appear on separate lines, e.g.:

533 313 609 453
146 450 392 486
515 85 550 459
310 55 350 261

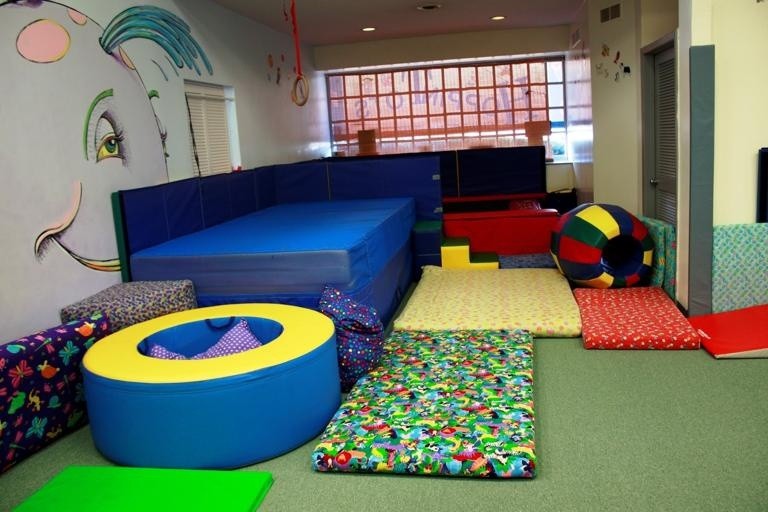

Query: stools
59 278 199 334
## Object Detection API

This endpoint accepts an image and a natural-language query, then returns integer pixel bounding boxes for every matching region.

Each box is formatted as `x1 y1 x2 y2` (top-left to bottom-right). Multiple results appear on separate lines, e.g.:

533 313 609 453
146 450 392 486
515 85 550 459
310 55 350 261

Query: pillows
316 282 385 391
147 317 263 359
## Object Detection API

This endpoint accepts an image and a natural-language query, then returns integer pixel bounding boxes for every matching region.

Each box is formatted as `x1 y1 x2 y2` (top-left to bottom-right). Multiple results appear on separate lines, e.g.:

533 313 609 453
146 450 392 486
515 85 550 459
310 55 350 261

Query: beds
129 196 418 334
